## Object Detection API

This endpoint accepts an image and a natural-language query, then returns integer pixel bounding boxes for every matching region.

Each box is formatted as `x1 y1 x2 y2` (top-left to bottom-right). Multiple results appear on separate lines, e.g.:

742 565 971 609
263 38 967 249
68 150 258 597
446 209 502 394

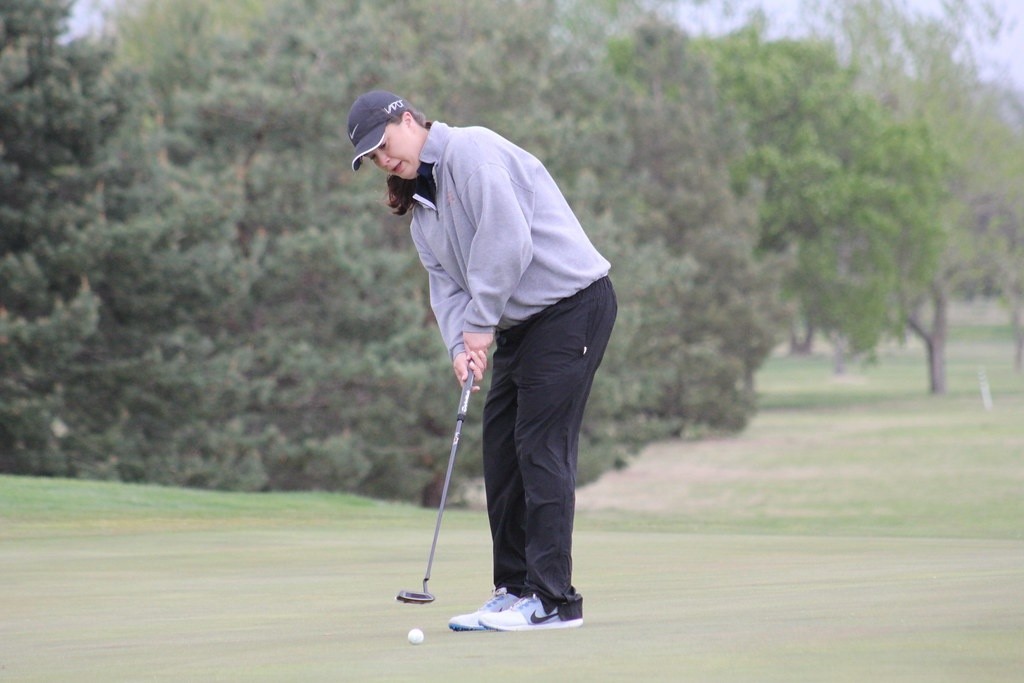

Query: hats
349 91 405 171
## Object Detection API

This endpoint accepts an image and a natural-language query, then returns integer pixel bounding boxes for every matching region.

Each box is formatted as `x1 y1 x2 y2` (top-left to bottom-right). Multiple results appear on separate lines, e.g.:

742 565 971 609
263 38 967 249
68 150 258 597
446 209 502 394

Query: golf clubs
394 357 476 605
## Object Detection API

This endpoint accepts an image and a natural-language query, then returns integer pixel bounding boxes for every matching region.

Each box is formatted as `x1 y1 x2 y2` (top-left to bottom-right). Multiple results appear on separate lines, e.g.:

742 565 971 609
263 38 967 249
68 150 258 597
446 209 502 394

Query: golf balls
407 628 424 645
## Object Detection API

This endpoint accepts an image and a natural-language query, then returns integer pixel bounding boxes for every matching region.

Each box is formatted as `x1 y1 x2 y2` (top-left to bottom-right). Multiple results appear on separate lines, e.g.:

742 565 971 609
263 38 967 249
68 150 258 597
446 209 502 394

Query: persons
347 89 618 633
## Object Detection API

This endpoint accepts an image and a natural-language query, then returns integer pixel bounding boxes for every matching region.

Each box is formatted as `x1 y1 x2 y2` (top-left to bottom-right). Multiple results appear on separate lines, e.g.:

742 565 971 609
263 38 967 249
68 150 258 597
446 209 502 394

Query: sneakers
478 593 583 630
448 586 522 629
